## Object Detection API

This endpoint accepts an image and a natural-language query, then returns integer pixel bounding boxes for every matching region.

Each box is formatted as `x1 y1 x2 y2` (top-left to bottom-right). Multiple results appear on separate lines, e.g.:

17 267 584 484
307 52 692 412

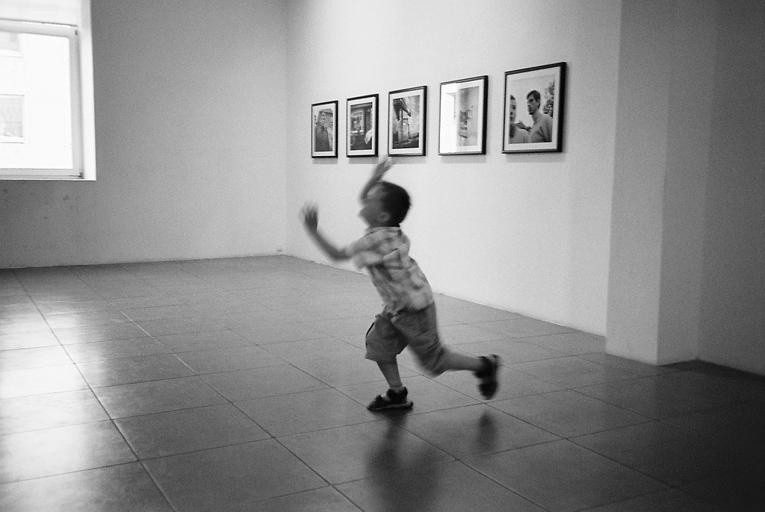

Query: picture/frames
310 61 567 160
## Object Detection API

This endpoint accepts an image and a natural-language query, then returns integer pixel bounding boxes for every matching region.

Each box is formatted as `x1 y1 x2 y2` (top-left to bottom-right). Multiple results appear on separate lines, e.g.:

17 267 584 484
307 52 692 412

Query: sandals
475 354 499 400
367 386 414 410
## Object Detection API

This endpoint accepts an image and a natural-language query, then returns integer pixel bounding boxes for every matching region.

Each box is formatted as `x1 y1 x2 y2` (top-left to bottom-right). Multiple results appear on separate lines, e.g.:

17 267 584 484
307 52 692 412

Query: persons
296 152 503 411
509 95 529 143
526 90 553 144
326 116 333 151
314 112 331 152
